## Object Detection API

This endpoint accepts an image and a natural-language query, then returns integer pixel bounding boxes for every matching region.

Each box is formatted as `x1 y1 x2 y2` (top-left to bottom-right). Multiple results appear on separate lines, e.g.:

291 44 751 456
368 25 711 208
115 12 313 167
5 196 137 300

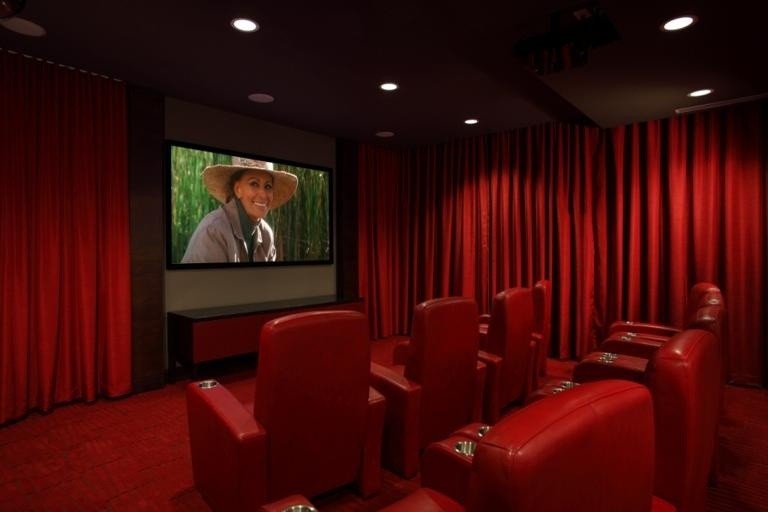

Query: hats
202 156 299 210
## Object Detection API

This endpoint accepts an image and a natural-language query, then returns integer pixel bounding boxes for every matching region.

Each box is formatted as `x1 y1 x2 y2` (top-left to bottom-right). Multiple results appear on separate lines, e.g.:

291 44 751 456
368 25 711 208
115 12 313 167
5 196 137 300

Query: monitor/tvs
162 139 335 271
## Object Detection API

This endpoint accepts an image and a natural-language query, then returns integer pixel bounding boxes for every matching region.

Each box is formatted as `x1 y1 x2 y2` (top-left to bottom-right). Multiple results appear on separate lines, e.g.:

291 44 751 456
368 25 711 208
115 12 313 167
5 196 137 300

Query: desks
166 295 365 386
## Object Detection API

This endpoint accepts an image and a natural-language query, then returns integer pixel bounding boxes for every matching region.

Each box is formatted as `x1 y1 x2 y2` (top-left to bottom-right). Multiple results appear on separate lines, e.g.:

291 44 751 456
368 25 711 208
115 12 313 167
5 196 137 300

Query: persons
174 152 301 265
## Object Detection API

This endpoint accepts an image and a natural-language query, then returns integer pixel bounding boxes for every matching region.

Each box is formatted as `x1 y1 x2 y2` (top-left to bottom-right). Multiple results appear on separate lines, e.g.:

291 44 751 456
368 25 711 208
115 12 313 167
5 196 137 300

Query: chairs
190 309 384 506
455 329 720 509
368 298 488 479
482 278 551 380
479 287 536 422
263 382 654 510
572 279 725 382
